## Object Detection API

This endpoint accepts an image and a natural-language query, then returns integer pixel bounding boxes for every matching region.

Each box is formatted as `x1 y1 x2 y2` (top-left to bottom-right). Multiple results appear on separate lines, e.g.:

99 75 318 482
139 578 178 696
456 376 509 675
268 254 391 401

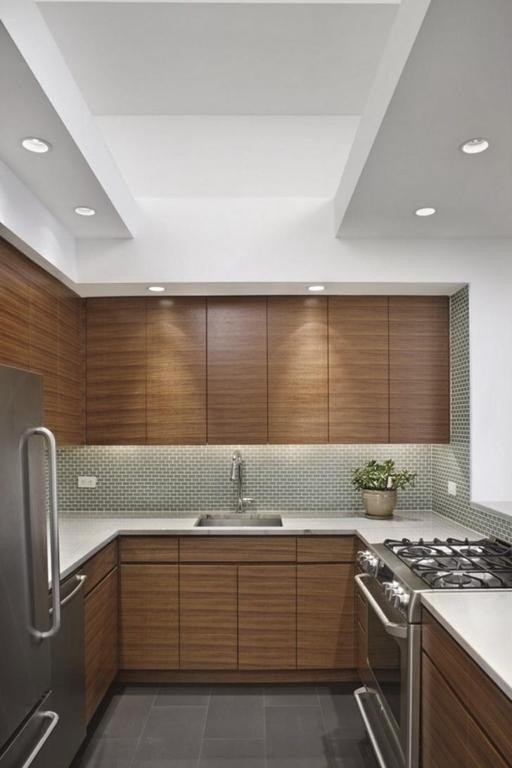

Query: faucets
229 449 242 500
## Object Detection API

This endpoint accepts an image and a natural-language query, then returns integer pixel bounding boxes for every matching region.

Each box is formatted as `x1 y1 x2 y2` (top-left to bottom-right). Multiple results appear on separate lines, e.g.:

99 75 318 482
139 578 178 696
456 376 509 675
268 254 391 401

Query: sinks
192 515 283 528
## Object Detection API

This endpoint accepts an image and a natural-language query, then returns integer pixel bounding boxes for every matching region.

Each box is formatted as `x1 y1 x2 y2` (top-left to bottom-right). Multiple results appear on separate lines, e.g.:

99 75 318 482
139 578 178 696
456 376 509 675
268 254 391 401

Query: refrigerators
1 362 61 766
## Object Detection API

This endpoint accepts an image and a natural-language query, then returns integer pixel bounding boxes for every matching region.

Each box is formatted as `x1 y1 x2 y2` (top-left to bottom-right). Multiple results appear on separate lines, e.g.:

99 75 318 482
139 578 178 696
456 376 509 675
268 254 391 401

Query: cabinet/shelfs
2 239 82 443
82 298 449 445
419 607 512 768
358 539 366 688
120 535 357 683
79 537 120 728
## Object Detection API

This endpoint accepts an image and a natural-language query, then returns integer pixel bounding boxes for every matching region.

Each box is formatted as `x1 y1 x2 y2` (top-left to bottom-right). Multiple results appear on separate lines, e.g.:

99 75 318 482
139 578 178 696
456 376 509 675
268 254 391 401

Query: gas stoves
357 535 512 623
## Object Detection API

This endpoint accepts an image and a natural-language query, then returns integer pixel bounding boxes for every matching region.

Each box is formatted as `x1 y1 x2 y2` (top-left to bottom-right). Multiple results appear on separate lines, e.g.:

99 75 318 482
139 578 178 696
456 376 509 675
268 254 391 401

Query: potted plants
350 457 418 520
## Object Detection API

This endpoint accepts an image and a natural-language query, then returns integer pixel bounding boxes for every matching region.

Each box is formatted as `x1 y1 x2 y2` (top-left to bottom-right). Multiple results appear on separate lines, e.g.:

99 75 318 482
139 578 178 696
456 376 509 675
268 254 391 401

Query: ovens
347 569 421 767
